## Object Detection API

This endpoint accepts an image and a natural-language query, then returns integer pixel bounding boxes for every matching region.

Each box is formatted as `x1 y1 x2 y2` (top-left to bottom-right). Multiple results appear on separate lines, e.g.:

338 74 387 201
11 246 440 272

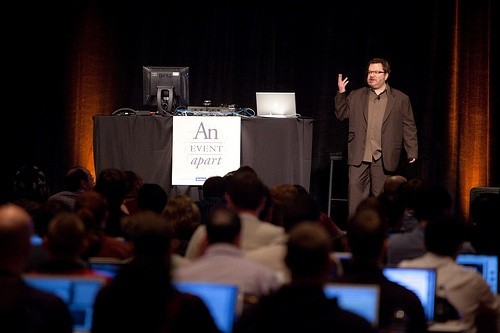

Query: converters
135 111 151 116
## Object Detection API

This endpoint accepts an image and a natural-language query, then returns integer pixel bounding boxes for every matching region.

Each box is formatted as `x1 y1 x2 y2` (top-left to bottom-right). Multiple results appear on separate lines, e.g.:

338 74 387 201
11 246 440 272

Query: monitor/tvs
170 281 221 333
90 256 123 280
455 253 498 296
322 282 380 329
22 273 109 333
143 66 189 107
383 267 436 324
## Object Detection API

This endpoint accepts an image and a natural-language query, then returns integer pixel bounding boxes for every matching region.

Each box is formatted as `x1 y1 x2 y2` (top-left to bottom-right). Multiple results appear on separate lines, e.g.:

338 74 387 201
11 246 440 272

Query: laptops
256 92 298 118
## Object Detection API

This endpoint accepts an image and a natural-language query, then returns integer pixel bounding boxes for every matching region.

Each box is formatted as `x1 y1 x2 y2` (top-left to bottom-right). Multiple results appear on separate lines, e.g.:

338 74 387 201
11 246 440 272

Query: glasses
366 70 386 74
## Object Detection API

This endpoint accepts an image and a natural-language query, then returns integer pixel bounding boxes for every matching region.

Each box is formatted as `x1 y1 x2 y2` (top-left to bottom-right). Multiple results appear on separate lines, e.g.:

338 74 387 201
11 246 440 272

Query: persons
332 225 430 333
176 204 286 332
89 225 226 333
397 213 499 333
232 222 375 333
334 57 419 226
24 212 115 296
0 202 75 333
1 150 500 268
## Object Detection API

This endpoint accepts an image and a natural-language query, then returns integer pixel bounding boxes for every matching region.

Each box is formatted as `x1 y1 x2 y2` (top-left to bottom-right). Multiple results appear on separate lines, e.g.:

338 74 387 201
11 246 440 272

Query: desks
91 115 314 200
